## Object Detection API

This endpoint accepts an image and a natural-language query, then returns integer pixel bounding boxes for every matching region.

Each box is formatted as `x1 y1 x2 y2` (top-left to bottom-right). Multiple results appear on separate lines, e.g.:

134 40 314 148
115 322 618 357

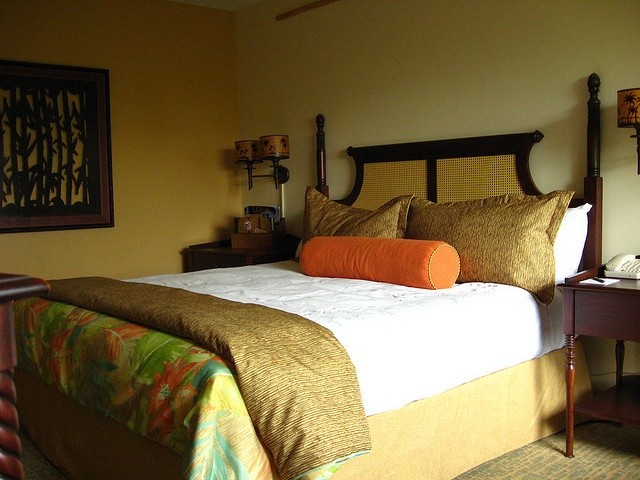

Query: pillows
408 191 573 304
546 203 594 283
302 238 460 290
302 186 413 241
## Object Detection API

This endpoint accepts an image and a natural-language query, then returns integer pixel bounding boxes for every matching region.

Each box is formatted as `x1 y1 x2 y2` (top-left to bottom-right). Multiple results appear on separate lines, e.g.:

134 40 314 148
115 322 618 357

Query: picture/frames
0 58 115 230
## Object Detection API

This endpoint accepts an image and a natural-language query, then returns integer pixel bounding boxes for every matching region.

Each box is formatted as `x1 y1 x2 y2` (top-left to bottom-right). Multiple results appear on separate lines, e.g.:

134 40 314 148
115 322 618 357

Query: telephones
604 253 640 279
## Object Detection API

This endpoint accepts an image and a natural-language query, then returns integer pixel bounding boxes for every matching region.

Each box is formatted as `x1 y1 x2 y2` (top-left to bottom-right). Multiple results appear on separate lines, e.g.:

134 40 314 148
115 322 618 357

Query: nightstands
553 262 640 457
186 233 299 272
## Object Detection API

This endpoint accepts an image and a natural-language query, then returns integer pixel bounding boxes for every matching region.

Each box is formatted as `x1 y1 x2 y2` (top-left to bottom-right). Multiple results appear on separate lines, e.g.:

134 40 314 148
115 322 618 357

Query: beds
19 73 603 480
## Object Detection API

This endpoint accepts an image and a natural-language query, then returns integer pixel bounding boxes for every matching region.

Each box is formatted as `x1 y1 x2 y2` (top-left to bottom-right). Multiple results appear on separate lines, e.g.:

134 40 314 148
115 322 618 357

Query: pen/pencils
593 276 605 281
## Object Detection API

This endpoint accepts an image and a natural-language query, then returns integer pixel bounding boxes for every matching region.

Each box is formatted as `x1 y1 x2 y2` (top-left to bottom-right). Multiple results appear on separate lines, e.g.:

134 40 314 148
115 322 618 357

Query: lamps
233 135 290 188
617 88 639 175
